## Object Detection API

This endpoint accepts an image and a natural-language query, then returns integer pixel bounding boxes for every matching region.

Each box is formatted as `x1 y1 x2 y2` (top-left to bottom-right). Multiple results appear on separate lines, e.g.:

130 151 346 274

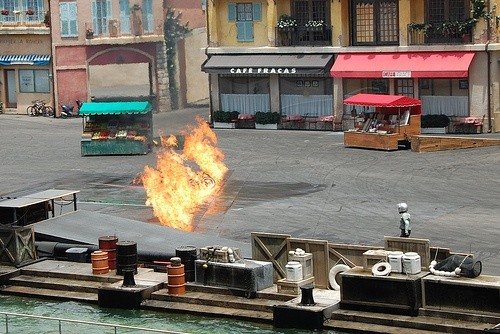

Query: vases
287 27 295 31
279 27 286 31
28 13 34 15
307 27 314 30
315 27 323 31
2 12 9 15
462 34 471 42
86 36 93 39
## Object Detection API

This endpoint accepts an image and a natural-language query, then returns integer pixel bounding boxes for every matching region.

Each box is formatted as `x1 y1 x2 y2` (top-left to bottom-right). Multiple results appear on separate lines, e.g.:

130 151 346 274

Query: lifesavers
329 265 350 290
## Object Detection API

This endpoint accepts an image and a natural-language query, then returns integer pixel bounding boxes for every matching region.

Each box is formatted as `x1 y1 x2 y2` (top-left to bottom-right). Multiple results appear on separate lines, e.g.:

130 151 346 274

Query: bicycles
26 99 55 118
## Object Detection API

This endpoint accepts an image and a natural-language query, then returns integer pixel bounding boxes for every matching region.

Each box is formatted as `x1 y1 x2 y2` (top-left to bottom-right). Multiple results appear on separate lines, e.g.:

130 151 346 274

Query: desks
0 188 81 226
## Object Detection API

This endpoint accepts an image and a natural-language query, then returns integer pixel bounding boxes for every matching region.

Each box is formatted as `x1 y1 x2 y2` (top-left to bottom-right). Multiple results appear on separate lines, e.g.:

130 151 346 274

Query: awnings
0 54 51 65
204 54 332 73
331 51 477 78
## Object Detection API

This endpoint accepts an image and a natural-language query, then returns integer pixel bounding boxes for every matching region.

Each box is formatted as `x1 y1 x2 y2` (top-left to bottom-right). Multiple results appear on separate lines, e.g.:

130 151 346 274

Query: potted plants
211 111 240 129
253 111 281 130
421 114 450 134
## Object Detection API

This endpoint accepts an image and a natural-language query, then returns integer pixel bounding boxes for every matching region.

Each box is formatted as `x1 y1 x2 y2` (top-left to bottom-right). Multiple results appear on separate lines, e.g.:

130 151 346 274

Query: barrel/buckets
91 252 109 274
116 240 137 275
167 264 185 295
99 236 118 270
175 245 197 282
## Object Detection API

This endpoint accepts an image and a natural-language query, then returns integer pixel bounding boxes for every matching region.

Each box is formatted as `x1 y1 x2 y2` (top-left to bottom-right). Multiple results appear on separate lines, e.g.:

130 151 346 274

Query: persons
396 203 412 237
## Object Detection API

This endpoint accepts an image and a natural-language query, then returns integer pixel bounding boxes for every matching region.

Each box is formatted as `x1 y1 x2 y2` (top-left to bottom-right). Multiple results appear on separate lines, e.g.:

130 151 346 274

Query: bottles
389 251 421 275
285 262 302 282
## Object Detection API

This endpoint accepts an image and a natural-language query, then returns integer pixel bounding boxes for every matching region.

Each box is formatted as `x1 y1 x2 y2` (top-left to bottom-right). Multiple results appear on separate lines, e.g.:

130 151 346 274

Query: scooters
59 98 82 119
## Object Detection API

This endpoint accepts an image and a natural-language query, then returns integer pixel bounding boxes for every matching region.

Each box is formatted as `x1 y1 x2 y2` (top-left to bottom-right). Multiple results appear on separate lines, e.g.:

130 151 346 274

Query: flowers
277 18 328 28
86 27 94 36
1 9 33 15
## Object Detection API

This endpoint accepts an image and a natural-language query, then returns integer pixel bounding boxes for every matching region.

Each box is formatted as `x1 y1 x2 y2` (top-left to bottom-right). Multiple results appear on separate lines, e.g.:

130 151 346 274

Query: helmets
397 203 408 214
